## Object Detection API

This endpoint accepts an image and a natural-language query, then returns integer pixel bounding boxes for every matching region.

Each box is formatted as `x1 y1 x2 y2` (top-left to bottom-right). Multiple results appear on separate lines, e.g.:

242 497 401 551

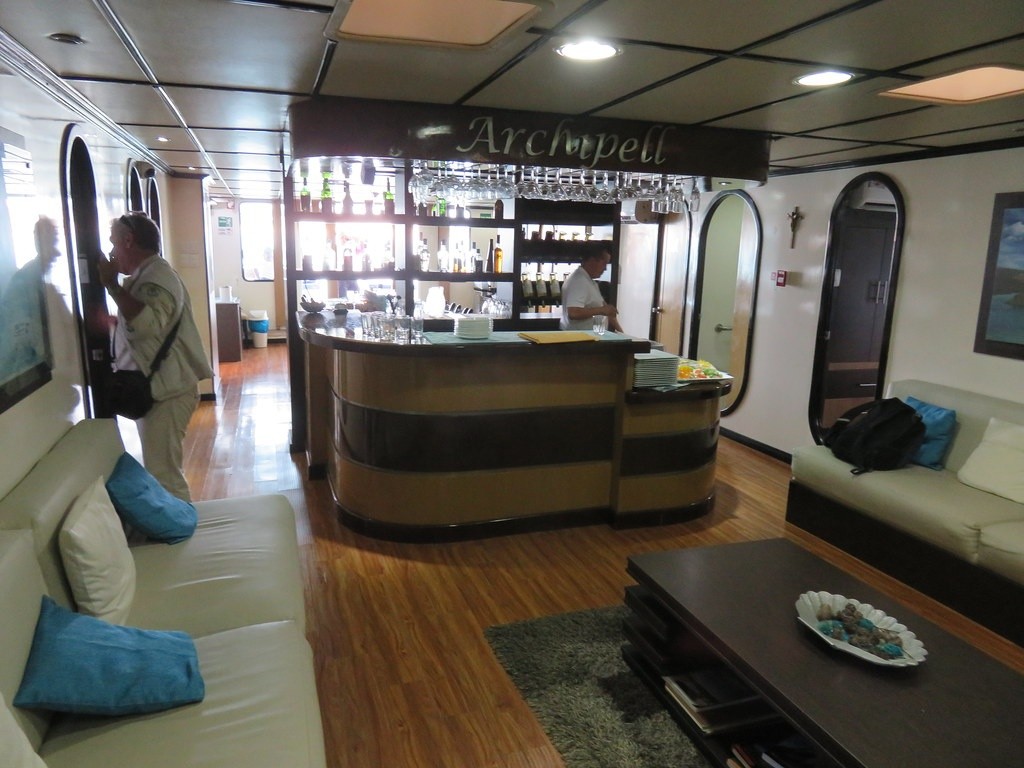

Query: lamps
360 157 376 186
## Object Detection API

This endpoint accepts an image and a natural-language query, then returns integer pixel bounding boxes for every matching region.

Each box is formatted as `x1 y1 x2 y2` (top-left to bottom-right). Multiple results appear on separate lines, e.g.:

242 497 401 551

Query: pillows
12 594 206 717
904 396 956 472
105 452 199 545
956 416 1024 504
58 474 138 628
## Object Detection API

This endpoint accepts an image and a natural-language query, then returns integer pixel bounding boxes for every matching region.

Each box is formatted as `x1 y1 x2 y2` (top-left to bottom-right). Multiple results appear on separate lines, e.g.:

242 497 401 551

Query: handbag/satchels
112 369 153 420
823 397 927 476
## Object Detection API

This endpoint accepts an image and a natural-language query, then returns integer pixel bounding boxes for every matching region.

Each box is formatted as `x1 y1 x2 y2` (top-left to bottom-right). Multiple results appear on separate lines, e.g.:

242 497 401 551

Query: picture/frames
973 191 1024 362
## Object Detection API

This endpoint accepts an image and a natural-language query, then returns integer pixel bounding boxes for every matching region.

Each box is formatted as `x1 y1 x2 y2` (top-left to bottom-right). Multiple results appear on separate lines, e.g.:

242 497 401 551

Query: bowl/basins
300 302 326 312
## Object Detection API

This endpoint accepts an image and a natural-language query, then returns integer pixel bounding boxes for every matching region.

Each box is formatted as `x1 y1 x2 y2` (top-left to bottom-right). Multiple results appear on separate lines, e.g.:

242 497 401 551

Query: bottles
343 183 353 214
532 231 594 266
321 178 332 213
495 199 503 220
414 232 502 274
522 272 571 308
385 185 394 215
300 178 311 212
320 239 395 274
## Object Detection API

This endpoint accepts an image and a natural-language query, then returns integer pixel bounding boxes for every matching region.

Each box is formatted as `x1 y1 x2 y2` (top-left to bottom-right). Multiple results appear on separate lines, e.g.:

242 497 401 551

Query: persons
559 245 624 333
95 211 216 504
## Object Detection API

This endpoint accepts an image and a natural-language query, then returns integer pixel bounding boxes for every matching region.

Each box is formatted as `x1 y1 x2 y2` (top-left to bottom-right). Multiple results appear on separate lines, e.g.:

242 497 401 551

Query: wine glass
407 159 700 215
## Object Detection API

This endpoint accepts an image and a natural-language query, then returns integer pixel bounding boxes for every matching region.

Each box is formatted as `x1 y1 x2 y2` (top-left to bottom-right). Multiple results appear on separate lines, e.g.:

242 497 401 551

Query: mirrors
807 170 906 447
681 189 763 421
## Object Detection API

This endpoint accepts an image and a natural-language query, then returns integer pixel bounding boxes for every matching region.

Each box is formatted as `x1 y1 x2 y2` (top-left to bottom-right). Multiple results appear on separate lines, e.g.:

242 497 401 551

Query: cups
481 298 512 319
360 311 424 343
593 315 607 334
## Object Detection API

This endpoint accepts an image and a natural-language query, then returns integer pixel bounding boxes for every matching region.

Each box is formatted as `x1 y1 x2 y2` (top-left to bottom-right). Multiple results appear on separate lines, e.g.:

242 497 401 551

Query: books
517 330 596 344
661 671 826 768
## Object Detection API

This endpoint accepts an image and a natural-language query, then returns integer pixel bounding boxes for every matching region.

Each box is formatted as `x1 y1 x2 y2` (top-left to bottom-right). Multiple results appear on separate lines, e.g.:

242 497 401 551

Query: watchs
108 286 120 296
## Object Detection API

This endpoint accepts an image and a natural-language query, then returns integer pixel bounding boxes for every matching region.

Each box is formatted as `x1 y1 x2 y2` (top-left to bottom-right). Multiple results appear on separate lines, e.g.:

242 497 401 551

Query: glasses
119 215 136 236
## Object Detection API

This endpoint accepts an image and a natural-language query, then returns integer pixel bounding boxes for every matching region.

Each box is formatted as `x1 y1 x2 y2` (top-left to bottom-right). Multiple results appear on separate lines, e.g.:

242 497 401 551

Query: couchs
787 378 1024 648
0 419 332 768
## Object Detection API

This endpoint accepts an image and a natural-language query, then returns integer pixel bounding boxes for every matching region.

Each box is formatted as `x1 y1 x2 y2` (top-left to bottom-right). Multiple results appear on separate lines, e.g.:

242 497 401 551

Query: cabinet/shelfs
281 177 623 330
216 297 242 362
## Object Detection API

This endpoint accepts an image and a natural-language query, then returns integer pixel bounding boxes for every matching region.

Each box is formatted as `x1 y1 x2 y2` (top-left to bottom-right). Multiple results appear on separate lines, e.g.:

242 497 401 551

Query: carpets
483 603 729 768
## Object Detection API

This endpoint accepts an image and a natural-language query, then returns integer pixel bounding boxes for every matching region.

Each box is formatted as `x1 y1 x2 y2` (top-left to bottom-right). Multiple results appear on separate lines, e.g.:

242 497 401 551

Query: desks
620 536 1024 768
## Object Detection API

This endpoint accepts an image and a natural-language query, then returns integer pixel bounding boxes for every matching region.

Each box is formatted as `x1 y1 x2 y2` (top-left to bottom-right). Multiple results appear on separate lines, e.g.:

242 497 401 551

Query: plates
453 314 494 339
795 590 928 665
632 348 734 388
